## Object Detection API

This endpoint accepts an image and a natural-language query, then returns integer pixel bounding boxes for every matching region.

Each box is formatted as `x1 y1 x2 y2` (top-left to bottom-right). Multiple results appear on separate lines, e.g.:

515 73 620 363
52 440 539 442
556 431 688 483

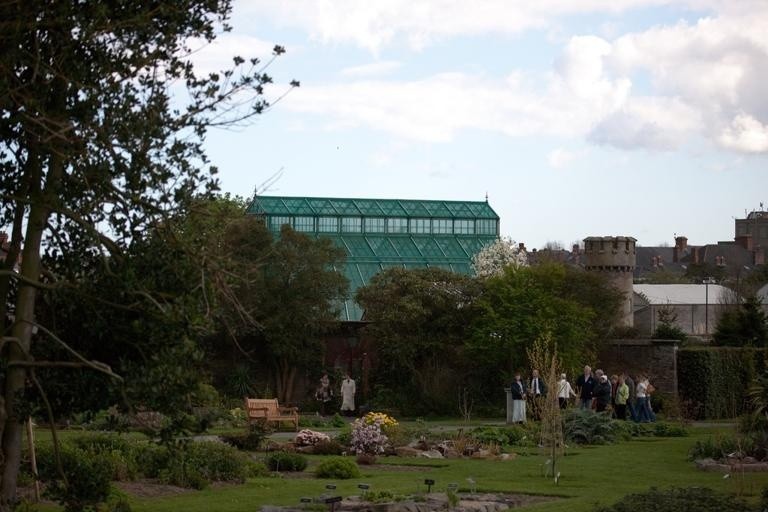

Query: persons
528 368 546 423
573 365 658 422
340 370 357 416
314 369 335 420
511 370 528 424
554 373 578 410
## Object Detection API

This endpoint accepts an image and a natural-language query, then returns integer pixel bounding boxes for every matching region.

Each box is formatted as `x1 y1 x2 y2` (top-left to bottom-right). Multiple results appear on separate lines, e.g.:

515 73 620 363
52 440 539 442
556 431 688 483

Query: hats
600 374 608 381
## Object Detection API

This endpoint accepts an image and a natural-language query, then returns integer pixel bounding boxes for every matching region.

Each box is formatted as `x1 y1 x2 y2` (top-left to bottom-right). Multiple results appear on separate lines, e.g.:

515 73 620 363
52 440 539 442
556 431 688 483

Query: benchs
245 397 299 432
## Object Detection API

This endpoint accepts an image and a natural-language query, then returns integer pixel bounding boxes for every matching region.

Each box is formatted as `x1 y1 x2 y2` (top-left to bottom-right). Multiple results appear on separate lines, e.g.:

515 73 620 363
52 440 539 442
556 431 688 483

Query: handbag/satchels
646 385 656 394
592 397 597 409
